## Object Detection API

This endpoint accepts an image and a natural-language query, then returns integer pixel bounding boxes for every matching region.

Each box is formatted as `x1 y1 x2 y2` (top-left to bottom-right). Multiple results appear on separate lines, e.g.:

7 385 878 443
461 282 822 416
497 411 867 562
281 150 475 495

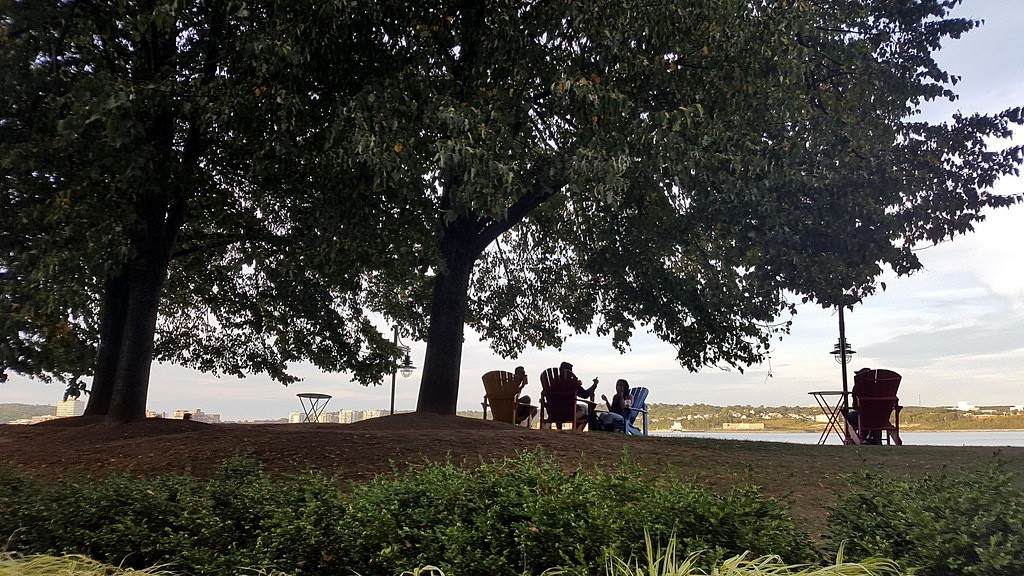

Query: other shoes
573 430 584 435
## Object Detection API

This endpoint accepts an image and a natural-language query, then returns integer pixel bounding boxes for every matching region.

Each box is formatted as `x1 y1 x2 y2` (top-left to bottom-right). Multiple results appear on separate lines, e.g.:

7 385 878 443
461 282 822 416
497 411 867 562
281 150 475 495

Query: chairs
841 368 904 445
539 366 598 429
480 371 539 427
595 387 650 435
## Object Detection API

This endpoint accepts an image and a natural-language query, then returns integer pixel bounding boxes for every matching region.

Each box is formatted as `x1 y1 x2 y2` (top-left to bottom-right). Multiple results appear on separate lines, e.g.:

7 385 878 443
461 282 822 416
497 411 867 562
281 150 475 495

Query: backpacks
595 409 624 433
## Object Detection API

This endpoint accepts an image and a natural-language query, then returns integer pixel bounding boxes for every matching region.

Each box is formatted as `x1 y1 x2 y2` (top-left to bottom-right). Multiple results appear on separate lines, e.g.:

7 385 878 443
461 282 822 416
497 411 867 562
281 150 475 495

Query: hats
561 362 573 371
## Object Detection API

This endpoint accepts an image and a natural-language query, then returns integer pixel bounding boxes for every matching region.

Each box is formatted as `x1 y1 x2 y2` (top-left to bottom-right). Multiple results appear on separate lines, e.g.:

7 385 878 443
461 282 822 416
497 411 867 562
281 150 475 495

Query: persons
553 362 599 431
515 367 537 427
852 367 873 444
598 379 634 431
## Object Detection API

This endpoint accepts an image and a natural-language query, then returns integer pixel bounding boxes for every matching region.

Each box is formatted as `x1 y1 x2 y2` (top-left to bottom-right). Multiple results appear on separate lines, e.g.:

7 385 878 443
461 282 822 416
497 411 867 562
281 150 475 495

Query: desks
296 393 332 424
807 390 853 445
